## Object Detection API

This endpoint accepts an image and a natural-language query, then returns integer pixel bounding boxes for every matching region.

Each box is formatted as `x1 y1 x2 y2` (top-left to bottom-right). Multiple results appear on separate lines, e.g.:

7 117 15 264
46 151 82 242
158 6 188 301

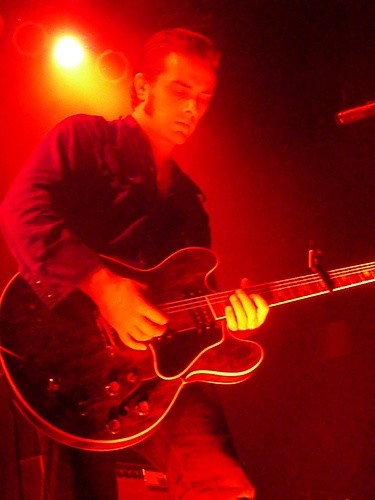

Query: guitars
0 246 375 453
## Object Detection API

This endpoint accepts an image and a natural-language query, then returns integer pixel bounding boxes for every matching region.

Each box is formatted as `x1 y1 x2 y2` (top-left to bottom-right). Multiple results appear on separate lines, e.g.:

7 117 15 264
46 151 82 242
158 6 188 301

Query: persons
11 26 269 500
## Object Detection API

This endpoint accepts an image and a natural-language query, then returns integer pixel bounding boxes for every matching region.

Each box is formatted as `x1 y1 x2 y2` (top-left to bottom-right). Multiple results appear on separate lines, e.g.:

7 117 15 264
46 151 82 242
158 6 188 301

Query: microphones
336 102 375 126
